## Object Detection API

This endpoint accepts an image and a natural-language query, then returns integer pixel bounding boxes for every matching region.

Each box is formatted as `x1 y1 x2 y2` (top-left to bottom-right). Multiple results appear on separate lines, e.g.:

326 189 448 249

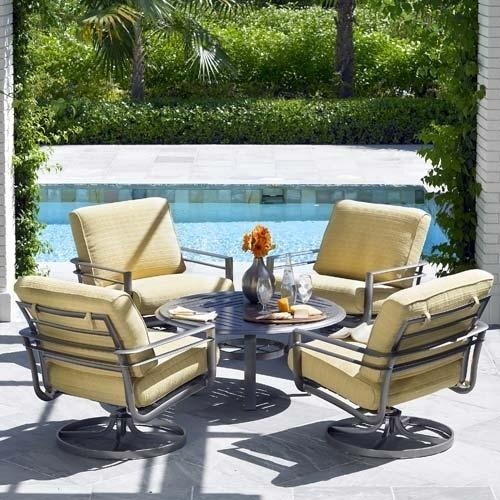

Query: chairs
14 274 222 462
68 195 234 334
264 198 432 335
285 267 495 459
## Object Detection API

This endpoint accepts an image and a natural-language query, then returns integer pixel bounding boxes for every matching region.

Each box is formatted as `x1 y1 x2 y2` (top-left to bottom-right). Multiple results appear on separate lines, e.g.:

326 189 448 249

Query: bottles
280 252 297 304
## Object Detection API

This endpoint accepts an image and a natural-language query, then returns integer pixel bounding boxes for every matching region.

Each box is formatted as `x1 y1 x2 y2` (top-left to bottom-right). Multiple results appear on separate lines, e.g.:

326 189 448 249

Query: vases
241 256 277 306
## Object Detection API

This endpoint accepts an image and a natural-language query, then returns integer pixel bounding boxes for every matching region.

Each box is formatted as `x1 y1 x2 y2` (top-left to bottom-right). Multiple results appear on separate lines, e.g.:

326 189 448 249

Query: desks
155 289 348 413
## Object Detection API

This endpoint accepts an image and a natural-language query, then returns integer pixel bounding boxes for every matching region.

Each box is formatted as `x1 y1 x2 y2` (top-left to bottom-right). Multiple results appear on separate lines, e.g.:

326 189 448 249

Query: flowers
241 224 276 259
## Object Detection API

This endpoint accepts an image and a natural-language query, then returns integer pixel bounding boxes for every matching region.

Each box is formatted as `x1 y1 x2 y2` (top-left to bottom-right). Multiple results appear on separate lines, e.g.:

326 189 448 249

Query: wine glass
296 274 313 303
256 276 274 314
